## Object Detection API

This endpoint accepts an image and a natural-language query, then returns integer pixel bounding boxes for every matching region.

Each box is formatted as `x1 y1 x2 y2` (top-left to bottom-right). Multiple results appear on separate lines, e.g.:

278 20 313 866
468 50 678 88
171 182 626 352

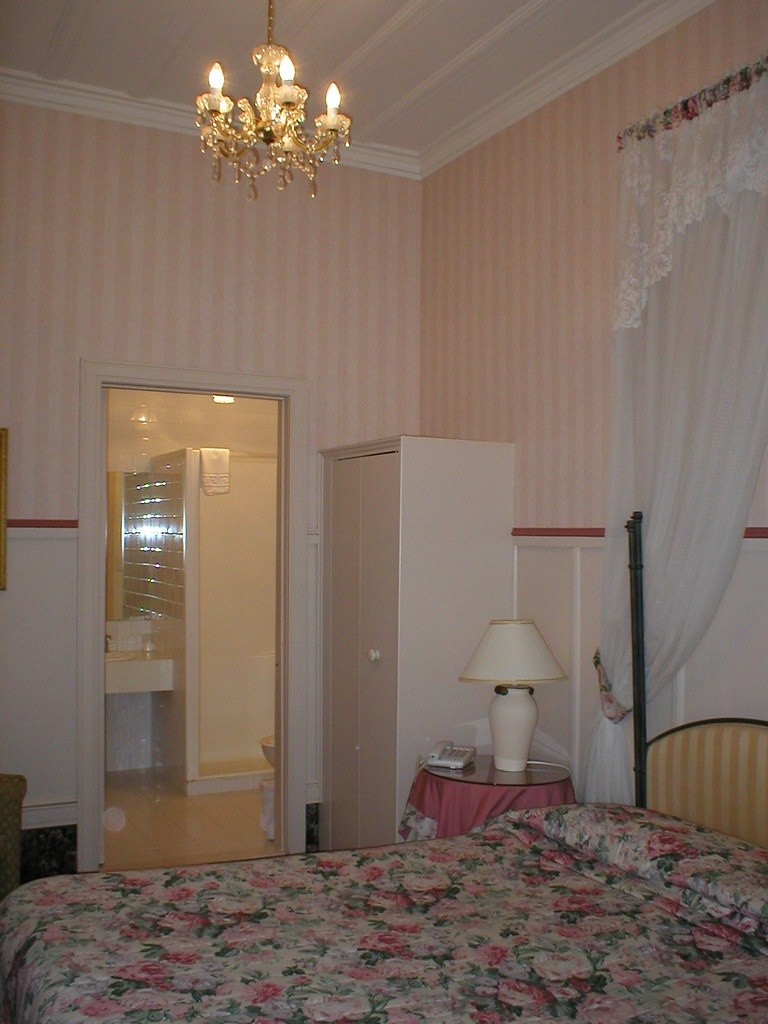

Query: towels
199 447 231 496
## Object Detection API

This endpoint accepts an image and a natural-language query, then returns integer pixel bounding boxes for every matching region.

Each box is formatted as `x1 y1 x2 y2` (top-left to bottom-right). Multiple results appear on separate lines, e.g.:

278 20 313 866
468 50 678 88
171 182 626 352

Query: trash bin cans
259 779 275 841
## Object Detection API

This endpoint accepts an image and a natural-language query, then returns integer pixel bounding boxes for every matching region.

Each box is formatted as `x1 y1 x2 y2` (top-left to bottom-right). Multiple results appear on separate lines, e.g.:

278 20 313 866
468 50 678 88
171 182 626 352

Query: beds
0 511 768 1024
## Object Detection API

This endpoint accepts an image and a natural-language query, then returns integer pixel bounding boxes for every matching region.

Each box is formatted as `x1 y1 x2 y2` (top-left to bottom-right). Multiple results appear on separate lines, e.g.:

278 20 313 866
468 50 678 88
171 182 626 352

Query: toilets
261 735 275 769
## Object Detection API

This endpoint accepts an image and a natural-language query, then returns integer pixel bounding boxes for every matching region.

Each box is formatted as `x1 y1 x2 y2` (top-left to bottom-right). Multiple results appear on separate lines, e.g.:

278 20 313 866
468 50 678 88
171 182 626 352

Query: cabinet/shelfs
319 434 515 851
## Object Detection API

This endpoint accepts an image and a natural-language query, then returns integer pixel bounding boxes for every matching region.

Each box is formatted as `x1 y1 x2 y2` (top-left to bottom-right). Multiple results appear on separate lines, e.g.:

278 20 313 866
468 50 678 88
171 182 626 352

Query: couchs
0 772 27 901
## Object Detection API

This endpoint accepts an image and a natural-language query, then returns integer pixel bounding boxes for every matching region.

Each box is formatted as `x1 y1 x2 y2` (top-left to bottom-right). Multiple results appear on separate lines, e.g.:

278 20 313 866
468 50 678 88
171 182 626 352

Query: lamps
194 0 352 203
458 619 569 772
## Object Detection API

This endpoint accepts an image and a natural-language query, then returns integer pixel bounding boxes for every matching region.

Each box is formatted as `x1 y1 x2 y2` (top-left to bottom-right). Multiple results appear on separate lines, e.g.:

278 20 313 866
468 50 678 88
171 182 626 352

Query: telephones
428 741 476 769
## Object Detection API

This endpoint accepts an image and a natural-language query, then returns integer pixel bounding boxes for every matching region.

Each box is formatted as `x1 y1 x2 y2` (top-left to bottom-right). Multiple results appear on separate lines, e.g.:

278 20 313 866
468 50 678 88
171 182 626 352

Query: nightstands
398 754 578 843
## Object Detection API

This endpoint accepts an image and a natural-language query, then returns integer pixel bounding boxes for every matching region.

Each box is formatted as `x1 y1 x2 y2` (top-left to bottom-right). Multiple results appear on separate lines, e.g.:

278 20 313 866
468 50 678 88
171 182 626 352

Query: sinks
106 651 136 662
